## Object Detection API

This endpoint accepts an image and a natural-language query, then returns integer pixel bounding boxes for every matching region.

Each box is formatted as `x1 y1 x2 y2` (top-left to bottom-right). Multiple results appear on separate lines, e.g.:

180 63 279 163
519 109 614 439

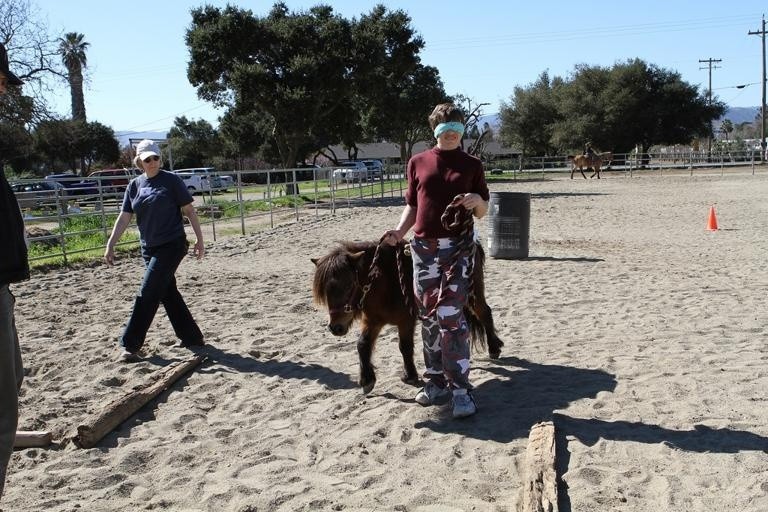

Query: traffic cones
706 206 720 231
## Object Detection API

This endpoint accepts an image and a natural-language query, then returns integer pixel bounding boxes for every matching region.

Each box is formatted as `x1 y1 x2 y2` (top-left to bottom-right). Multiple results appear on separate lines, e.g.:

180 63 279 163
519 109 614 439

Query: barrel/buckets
488 190 531 259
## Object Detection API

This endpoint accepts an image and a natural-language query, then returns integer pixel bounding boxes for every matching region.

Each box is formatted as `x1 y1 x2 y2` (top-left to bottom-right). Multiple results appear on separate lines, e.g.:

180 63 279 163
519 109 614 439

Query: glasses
141 157 160 162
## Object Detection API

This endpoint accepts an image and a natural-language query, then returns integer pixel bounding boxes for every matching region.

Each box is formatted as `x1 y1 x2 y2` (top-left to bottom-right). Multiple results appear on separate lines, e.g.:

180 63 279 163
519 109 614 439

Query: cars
11 167 234 213
333 161 368 182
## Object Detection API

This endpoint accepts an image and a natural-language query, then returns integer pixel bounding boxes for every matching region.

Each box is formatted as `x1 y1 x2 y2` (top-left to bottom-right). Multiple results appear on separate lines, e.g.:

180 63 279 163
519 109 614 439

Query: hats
135 138 162 163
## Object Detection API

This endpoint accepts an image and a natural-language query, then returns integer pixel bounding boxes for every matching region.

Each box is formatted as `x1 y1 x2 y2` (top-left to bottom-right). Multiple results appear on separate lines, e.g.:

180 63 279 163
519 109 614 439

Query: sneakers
449 385 480 420
414 377 449 404
170 340 198 351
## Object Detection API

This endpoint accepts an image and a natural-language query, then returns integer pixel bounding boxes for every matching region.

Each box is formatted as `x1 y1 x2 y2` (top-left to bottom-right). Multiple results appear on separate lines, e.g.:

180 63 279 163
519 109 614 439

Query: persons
0 165 31 512
584 133 601 162
105 140 207 354
379 102 491 417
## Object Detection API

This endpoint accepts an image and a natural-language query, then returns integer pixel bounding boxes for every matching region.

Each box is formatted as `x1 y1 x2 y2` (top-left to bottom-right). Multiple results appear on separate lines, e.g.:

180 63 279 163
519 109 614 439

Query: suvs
298 165 323 180
362 160 384 182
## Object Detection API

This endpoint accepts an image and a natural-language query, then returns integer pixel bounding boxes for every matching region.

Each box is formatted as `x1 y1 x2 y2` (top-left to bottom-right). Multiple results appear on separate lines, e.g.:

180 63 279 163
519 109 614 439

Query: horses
311 237 503 396
567 152 615 181
